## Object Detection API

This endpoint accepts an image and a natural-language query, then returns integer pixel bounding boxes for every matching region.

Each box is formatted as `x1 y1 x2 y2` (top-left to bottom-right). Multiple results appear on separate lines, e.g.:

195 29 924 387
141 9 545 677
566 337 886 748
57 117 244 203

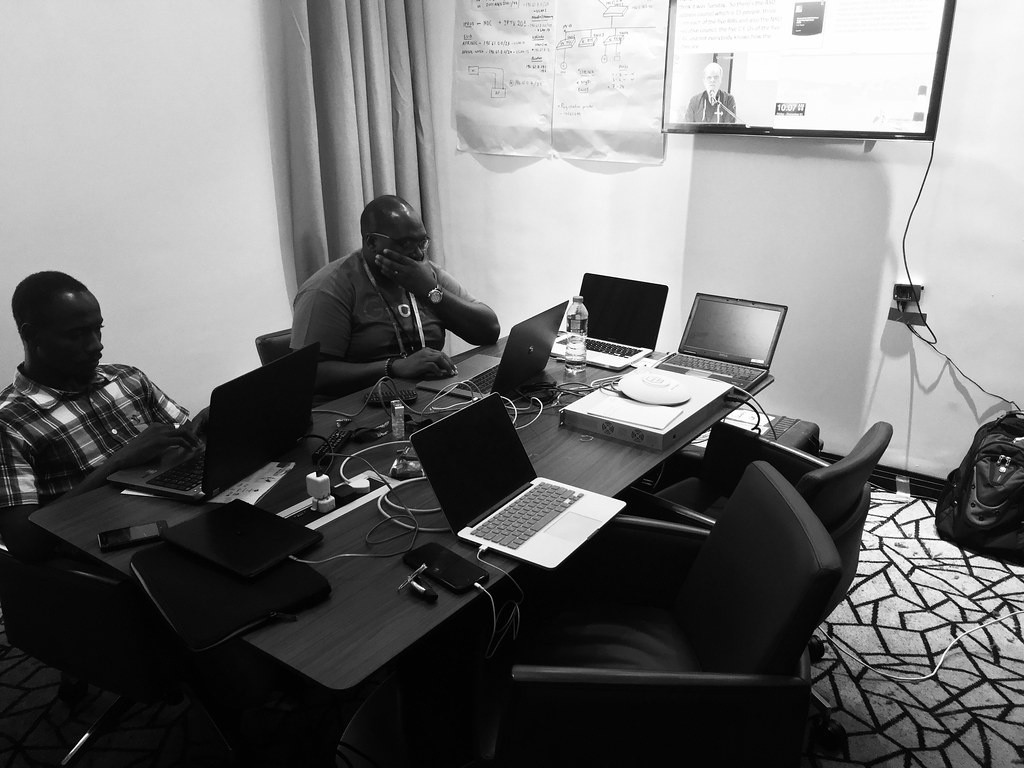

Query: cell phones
97 520 167 552
403 541 489 594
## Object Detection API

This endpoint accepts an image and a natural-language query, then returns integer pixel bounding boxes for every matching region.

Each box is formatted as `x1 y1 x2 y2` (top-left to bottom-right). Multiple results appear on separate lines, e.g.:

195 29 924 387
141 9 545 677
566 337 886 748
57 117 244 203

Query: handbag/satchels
129 541 333 656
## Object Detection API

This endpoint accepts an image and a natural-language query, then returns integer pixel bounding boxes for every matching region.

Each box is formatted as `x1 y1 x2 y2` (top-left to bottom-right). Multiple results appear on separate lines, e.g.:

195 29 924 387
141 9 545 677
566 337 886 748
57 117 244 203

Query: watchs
422 282 444 308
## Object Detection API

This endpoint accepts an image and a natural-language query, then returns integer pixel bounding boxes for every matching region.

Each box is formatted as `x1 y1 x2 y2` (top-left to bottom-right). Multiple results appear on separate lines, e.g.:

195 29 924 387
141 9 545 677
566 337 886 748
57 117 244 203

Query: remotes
312 428 352 463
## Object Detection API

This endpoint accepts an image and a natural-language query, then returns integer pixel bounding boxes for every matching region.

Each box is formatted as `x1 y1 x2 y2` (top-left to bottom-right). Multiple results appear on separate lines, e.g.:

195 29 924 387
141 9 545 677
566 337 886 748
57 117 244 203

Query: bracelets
384 356 401 378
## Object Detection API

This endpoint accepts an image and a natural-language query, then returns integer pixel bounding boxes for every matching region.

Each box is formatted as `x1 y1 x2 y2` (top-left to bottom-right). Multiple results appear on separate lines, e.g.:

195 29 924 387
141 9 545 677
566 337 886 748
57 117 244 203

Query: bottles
565 296 588 373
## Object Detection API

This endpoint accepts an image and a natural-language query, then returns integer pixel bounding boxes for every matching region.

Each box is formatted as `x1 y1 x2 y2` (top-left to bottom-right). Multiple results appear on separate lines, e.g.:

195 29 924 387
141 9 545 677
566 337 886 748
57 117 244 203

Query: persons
289 193 502 397
685 63 735 124
0 270 343 767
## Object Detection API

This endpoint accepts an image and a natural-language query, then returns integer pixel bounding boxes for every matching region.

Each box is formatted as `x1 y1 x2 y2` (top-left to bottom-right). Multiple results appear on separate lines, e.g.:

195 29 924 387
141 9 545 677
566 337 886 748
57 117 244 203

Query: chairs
0 534 180 711
475 458 843 768
624 419 894 664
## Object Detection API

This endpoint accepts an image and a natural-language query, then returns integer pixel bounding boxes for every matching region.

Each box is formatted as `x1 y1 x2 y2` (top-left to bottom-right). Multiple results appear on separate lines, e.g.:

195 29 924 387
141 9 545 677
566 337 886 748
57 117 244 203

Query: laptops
409 393 627 570
416 300 570 400
159 499 323 580
654 293 788 393
550 273 668 371
105 342 320 503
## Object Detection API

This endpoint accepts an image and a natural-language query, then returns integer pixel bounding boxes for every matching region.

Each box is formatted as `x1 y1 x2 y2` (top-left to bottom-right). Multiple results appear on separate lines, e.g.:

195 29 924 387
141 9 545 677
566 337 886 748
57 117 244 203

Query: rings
393 270 398 278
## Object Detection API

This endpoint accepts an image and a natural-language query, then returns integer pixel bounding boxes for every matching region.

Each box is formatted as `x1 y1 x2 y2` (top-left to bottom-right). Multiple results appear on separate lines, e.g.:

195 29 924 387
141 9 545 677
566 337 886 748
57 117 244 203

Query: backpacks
934 407 1024 566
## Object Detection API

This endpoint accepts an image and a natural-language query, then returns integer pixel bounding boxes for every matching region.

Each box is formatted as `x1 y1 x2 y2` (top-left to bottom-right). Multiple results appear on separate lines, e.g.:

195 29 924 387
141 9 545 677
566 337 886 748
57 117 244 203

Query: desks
25 331 775 768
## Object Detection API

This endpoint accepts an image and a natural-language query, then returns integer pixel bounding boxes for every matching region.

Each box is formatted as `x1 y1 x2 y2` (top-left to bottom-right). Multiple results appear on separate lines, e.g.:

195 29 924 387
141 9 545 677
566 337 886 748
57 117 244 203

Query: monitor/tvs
660 0 957 140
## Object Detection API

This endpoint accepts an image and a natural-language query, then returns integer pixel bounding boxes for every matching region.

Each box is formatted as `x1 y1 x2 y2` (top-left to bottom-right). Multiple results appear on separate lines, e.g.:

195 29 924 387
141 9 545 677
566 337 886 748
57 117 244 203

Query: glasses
364 232 431 254
